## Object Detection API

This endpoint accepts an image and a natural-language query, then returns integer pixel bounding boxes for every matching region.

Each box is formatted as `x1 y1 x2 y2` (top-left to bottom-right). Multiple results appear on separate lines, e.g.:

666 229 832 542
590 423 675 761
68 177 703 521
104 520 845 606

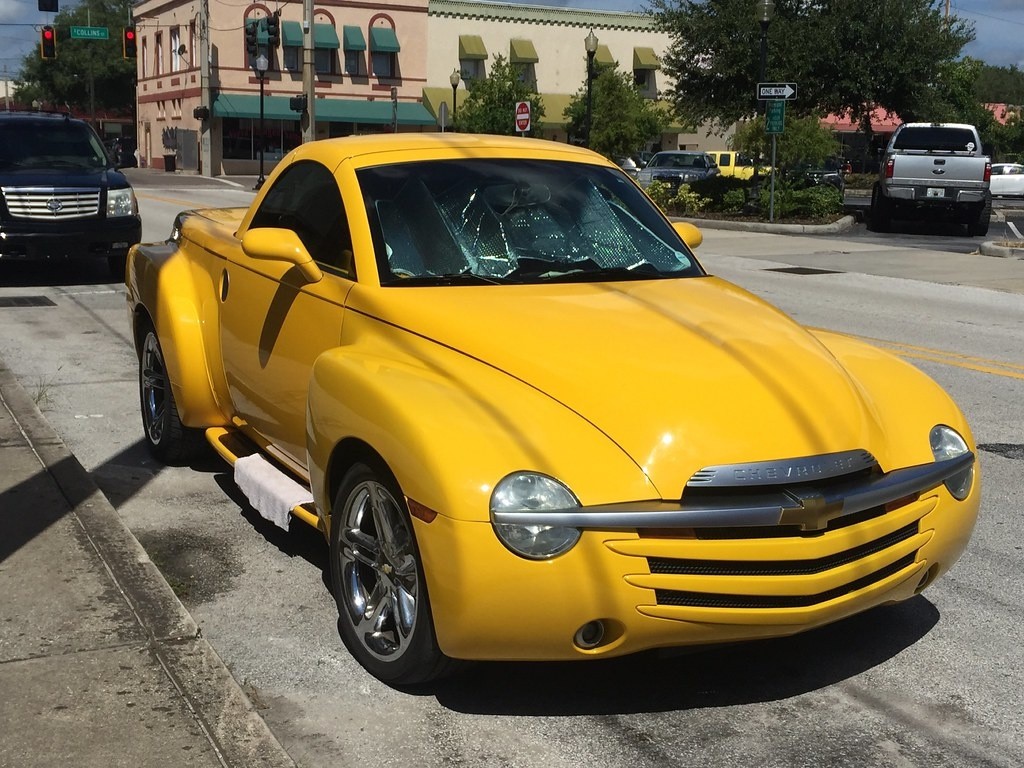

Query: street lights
449 67 461 128
252 55 270 190
584 28 598 150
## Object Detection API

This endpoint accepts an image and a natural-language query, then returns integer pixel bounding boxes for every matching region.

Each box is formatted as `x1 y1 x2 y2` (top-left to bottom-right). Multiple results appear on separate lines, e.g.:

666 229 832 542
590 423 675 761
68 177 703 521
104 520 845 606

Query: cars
610 156 638 178
631 149 722 190
632 154 647 169
127 132 980 688
989 163 1024 199
780 155 851 201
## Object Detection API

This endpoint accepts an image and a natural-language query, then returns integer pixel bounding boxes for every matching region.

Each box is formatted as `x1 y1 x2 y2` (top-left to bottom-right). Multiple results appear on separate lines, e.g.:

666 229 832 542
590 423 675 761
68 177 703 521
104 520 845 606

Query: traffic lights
123 26 138 60
40 26 58 61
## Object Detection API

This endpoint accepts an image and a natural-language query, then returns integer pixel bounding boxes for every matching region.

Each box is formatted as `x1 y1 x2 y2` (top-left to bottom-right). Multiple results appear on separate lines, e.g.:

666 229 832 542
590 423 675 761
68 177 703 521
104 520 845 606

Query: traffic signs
758 84 799 99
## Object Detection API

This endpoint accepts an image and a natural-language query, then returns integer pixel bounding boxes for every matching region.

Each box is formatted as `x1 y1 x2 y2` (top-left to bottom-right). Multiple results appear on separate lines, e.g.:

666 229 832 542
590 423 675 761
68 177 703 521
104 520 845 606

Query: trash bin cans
162 154 176 172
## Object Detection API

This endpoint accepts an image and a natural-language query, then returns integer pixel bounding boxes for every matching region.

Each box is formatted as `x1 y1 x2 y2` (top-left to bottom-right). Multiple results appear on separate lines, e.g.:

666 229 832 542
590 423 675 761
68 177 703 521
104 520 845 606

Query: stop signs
514 101 530 132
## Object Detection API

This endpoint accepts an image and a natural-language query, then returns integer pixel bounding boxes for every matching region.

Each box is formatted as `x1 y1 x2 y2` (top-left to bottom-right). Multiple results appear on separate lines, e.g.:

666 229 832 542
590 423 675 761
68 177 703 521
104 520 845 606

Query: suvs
0 109 144 271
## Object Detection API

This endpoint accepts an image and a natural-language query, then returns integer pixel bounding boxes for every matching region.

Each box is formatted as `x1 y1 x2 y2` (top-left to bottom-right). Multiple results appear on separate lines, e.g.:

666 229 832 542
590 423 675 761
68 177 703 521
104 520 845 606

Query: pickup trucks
703 151 778 181
867 122 993 237
